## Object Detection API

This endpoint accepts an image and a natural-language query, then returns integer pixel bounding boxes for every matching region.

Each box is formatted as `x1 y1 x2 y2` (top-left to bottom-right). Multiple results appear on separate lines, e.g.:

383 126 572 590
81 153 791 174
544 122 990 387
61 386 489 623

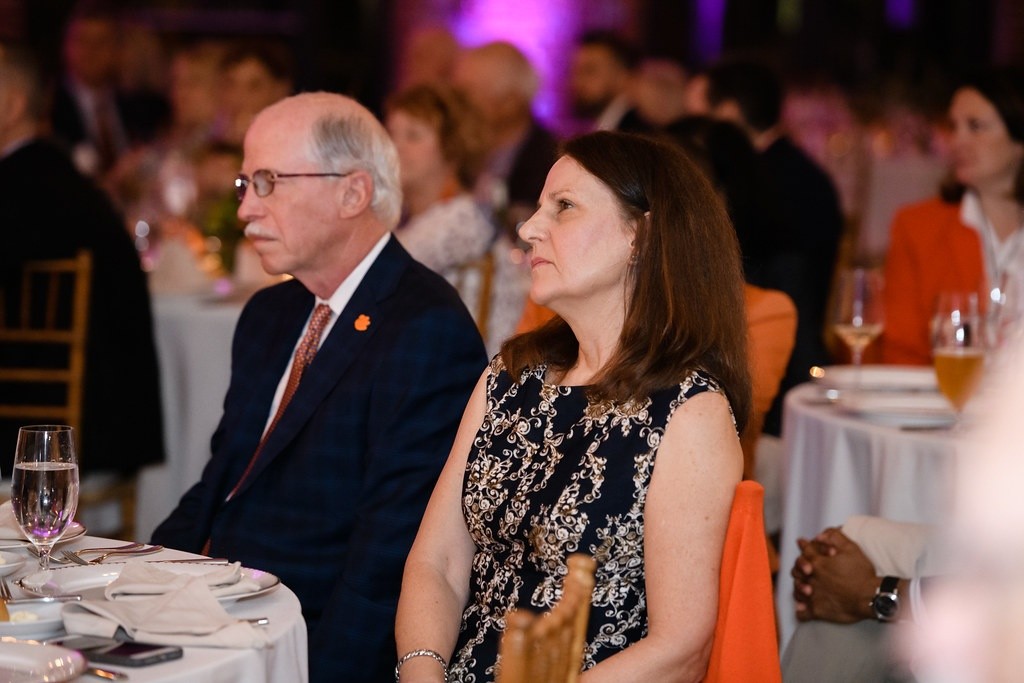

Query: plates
819 364 937 391
0 520 88 549
828 386 955 428
0 603 65 641
18 565 280 608
0 551 26 577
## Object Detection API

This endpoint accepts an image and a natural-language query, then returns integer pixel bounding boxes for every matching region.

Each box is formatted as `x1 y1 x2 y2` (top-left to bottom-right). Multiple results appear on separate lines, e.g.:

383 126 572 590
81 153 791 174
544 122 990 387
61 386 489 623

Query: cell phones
39 633 183 667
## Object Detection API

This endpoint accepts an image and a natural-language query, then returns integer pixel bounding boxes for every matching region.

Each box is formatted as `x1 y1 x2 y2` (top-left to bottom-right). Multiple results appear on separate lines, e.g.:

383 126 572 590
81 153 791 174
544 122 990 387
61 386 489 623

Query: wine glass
833 268 885 365
12 424 79 571
932 293 986 415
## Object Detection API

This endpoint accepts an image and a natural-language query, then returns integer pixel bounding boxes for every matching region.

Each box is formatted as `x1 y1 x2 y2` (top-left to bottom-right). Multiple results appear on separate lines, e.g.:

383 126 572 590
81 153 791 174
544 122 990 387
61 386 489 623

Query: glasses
234 169 346 199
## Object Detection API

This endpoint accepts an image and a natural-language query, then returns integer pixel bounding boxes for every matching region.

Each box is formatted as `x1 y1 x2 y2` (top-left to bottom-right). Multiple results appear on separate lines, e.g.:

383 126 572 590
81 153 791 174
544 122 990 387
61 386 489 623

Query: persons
395 130 753 683
450 40 560 241
107 24 297 215
563 27 632 132
386 79 463 219
688 61 845 437
615 39 743 176
0 39 168 481
146 93 487 683
883 68 1024 364
43 14 173 171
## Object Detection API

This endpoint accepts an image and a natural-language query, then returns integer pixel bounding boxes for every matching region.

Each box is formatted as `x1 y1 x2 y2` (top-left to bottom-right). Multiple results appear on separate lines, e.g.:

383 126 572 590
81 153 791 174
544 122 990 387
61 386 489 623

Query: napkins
0 500 28 539
863 410 953 431
106 557 261 602
61 579 265 649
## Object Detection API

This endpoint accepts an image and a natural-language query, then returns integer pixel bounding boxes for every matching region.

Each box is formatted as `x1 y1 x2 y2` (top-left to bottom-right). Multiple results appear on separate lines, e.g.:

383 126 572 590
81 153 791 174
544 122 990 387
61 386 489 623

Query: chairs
0 251 797 683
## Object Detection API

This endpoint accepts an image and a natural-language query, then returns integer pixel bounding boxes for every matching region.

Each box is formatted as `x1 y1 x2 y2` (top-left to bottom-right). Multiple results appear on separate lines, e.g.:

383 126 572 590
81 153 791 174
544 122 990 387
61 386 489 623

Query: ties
200 304 333 559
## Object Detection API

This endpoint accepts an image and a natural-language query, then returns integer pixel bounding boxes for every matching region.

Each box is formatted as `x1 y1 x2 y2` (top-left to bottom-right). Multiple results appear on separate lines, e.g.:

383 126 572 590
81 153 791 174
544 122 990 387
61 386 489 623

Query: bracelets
395 648 448 681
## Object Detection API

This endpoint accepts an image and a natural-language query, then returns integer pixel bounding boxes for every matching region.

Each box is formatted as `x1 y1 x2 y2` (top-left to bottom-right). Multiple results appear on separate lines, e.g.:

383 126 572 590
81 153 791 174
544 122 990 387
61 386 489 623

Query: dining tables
0 535 309 683
774 382 960 661
131 298 243 542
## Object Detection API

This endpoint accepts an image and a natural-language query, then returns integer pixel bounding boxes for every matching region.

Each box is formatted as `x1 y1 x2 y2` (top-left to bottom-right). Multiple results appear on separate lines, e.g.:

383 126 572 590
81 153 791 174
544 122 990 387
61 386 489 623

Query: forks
26 542 164 565
